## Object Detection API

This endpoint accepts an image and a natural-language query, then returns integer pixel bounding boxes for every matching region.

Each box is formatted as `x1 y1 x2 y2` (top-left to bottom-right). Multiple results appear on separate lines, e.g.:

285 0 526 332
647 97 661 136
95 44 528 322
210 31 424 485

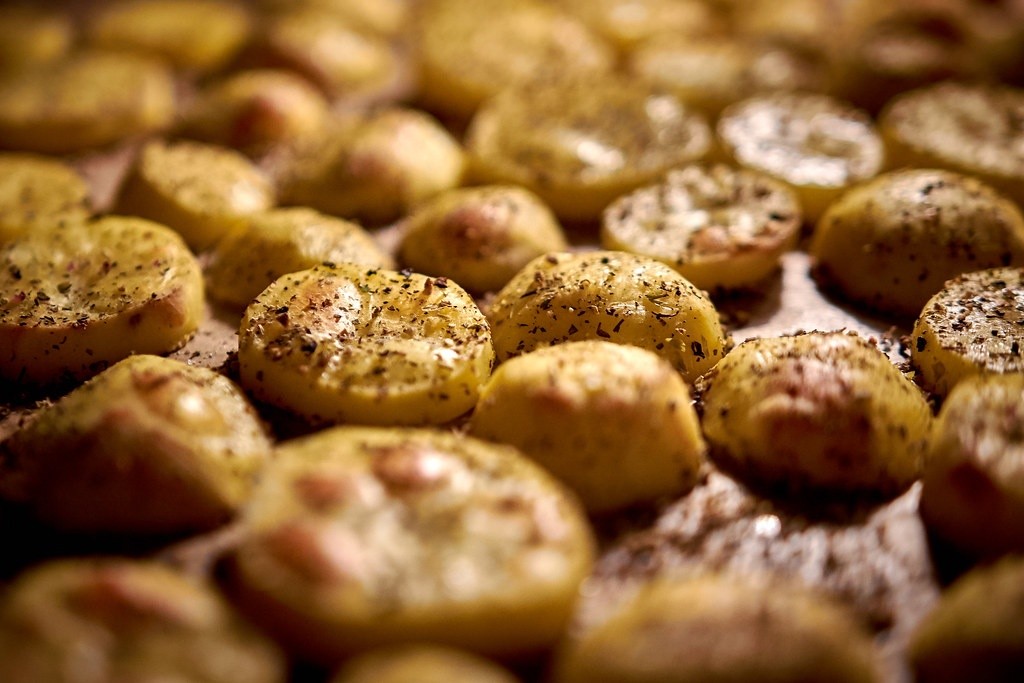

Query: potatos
0 0 1024 683
